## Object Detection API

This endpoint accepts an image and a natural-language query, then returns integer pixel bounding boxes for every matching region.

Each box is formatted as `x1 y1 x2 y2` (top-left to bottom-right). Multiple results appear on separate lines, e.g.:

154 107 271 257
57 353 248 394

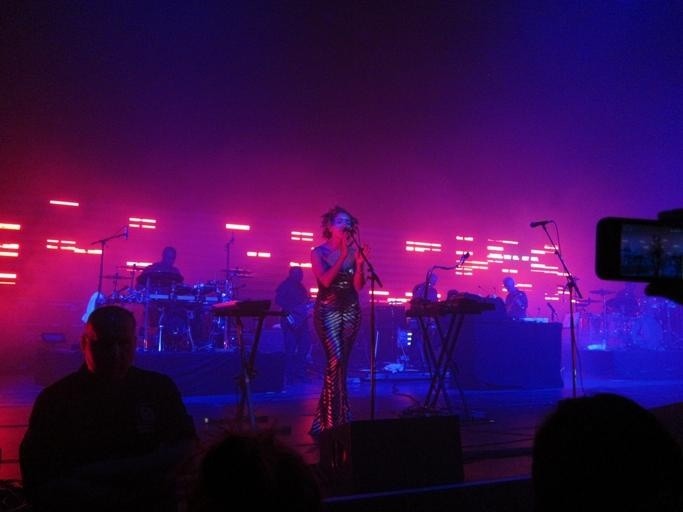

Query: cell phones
595 216 683 283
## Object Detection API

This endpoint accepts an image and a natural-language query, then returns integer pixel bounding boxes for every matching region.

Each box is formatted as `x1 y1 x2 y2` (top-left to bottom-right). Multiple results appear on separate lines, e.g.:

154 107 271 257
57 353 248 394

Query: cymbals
230 272 256 278
135 270 184 287
222 267 252 274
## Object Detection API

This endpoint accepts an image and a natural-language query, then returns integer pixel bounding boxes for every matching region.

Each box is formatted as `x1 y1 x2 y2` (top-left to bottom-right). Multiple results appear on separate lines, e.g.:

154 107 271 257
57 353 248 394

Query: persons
306 203 367 437
650 233 663 273
275 266 311 346
603 282 640 316
184 430 327 508
17 305 204 511
526 388 681 508
643 208 683 313
501 277 529 320
410 272 438 303
133 243 185 349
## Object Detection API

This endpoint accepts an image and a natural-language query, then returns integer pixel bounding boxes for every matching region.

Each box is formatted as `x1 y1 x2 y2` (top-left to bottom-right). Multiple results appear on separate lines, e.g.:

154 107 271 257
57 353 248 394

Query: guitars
285 300 318 332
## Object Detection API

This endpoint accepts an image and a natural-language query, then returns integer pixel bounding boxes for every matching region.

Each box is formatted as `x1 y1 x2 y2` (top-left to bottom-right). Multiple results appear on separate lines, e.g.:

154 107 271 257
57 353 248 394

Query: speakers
312 413 465 496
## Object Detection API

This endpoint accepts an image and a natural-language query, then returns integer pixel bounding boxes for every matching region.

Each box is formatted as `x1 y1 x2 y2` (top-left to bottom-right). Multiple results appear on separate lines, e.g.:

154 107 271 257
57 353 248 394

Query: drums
158 314 201 355
149 287 169 312
171 285 198 312
194 284 215 295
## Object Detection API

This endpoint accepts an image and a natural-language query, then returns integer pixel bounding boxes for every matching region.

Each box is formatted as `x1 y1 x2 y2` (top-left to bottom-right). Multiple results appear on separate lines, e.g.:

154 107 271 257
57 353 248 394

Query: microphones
530 220 551 228
460 253 470 265
343 226 356 233
547 303 557 316
224 239 233 249
124 227 128 240
